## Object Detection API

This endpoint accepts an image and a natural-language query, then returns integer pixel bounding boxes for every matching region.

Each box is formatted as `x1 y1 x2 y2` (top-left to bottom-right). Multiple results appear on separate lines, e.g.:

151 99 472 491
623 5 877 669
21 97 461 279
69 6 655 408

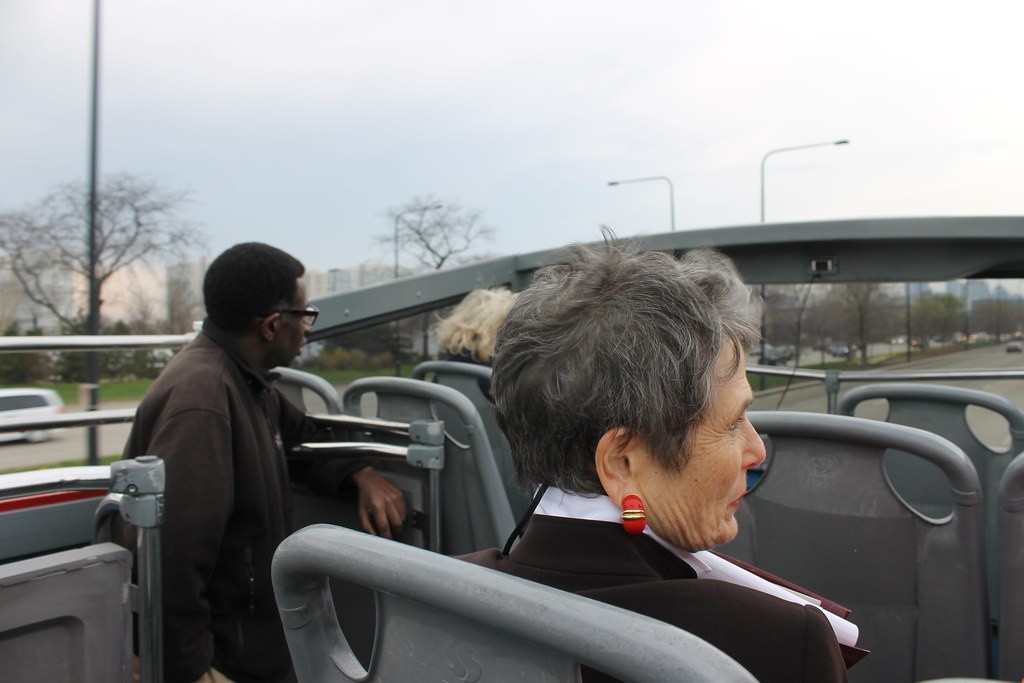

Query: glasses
274 304 321 328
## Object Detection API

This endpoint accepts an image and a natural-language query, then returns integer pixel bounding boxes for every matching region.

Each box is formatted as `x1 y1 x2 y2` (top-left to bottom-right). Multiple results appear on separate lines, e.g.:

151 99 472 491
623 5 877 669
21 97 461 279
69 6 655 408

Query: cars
749 342 797 367
892 330 991 351
814 336 858 358
1006 340 1024 354
0 388 66 440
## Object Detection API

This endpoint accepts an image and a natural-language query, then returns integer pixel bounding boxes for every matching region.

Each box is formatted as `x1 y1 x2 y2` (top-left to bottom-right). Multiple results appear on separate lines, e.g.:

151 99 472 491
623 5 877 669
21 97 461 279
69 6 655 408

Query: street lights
607 176 675 232
392 203 445 279
760 138 850 220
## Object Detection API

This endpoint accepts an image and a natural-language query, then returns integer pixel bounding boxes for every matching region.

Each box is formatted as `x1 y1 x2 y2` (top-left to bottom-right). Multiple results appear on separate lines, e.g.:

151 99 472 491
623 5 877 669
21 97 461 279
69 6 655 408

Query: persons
102 243 406 683
435 289 516 401
454 247 870 683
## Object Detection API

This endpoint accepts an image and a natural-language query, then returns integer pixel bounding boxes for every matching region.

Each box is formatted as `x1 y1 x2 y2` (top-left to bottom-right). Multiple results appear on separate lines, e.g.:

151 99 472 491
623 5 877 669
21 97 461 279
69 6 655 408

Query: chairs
268 361 1024 683
